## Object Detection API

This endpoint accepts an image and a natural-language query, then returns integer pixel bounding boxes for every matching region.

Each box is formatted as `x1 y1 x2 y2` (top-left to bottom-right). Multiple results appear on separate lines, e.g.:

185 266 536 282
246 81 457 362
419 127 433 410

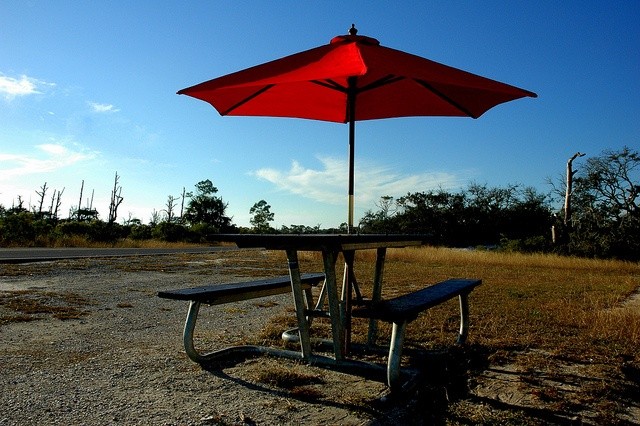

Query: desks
217 229 422 349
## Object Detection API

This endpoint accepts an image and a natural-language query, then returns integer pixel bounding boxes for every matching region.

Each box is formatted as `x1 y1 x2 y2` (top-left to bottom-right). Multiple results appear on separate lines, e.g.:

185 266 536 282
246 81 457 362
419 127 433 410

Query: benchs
352 277 482 388
158 273 325 365
